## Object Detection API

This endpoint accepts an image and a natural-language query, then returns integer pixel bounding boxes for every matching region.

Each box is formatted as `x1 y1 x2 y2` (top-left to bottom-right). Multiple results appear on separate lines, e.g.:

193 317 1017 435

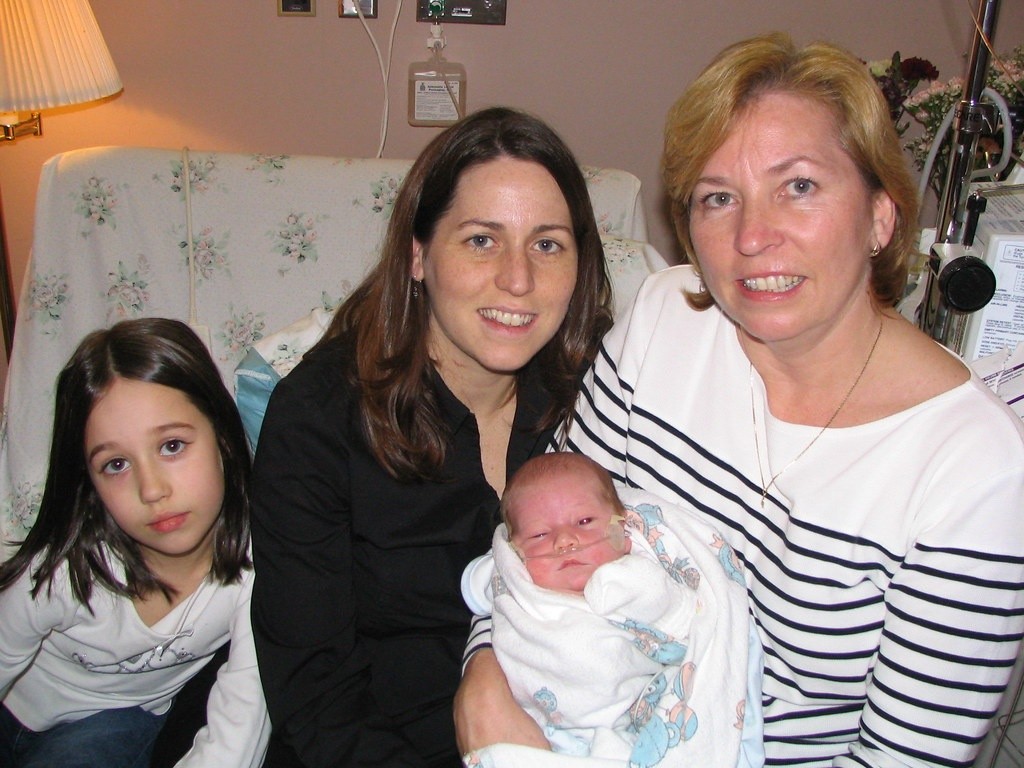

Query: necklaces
736 304 882 509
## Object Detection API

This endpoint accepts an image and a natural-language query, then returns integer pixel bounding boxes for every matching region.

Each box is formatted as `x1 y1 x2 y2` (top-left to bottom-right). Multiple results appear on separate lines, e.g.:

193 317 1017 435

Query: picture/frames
276 0 315 17
416 0 506 26
338 0 378 18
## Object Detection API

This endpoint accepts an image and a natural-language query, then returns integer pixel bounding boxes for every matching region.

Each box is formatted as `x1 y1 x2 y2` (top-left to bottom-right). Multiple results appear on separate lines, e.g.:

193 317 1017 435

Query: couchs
0 147 672 558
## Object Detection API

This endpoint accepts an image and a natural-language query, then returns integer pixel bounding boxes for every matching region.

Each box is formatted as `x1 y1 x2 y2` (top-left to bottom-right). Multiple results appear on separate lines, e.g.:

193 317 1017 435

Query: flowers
859 40 1024 212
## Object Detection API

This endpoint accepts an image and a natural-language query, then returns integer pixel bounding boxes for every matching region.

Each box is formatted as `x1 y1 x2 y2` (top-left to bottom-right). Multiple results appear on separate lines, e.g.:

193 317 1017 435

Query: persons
251 104 614 768
454 33 1024 768
490 452 767 768
0 317 273 768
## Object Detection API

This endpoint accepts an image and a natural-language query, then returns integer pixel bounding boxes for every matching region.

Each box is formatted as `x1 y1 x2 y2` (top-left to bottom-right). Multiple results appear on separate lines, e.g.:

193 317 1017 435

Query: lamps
0 0 123 143
